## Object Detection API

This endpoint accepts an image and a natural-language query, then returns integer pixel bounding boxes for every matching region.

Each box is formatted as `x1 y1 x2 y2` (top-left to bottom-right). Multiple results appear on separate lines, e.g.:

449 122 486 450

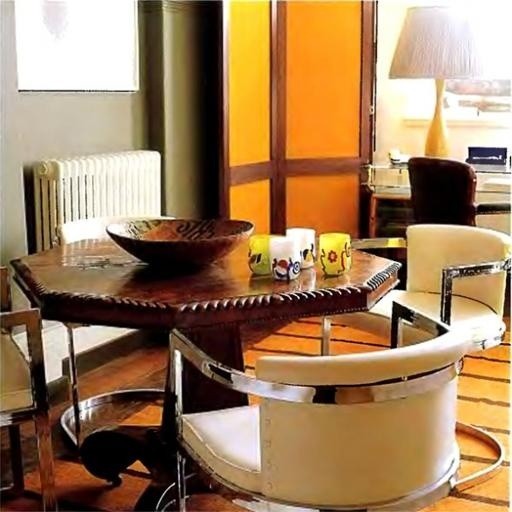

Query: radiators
33 149 163 253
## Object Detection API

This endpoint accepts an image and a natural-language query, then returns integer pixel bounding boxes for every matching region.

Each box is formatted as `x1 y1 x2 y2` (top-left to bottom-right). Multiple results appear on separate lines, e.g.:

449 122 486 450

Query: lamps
389 4 483 159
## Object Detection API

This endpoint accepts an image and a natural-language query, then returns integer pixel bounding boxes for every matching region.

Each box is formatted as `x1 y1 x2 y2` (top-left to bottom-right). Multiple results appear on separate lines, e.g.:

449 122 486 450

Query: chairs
406 156 510 228
0 267 60 511
163 320 474 510
392 225 512 491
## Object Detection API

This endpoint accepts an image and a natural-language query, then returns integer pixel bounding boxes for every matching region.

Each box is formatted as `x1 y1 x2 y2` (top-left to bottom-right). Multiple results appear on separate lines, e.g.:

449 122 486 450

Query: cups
248 227 351 282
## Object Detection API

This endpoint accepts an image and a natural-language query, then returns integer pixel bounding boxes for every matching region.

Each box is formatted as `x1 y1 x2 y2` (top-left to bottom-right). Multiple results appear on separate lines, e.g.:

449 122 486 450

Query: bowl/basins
105 218 255 275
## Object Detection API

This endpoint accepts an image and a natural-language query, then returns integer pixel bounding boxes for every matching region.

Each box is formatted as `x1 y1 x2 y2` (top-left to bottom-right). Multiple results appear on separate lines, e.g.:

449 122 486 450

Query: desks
363 185 511 259
